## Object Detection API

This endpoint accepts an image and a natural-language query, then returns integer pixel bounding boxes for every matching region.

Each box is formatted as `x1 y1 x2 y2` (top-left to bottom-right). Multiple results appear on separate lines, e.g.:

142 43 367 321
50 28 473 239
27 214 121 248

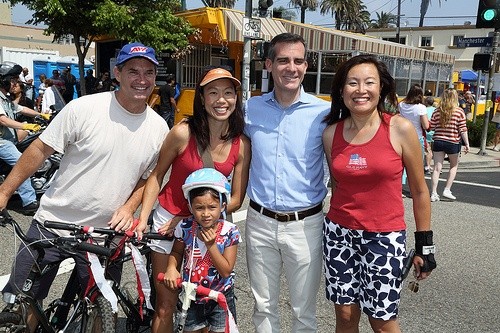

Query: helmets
181 167 231 206
0 61 24 88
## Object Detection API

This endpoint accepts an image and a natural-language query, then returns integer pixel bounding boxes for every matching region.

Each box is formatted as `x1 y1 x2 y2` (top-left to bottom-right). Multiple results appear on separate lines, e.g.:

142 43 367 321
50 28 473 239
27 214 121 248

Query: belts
249 198 323 222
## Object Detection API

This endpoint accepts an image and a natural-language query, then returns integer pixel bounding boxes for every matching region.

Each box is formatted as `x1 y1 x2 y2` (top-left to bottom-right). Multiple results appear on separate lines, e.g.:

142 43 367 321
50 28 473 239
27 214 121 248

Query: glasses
408 268 423 293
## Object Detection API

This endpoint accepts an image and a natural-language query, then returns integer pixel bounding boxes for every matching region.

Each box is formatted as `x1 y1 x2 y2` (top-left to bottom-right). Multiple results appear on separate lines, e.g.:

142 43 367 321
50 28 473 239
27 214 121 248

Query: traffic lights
476 0 500 29
472 52 493 71
258 0 273 12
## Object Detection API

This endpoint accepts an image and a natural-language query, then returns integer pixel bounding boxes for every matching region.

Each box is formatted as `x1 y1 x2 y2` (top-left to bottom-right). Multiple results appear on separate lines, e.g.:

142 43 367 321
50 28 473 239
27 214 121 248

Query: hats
199 67 241 88
115 43 160 66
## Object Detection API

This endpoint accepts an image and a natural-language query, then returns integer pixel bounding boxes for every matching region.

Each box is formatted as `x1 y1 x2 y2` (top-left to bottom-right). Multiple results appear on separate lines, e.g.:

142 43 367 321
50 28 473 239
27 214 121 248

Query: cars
475 86 500 103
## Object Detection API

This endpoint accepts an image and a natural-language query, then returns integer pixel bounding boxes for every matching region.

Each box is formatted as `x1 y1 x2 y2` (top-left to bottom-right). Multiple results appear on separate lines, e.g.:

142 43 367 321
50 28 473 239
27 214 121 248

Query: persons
0 42 171 333
457 88 487 116
424 88 433 96
163 168 242 333
130 67 252 333
424 96 443 175
322 53 437 333
430 88 472 202
0 61 56 213
397 84 429 198
175 33 342 333
50 66 120 105
150 73 180 130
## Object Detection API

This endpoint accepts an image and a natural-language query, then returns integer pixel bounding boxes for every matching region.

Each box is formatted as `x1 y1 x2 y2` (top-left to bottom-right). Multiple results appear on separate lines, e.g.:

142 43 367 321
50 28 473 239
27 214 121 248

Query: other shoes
401 190 413 199
20 200 40 215
442 189 457 200
431 192 441 202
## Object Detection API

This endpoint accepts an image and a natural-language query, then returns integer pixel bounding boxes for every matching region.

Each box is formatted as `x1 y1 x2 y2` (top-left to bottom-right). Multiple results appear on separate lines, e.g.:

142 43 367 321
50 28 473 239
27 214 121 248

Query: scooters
0 84 67 200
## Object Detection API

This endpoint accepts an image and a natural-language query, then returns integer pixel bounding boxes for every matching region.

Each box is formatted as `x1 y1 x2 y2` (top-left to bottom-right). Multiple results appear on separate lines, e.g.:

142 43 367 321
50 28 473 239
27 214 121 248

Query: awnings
224 11 288 43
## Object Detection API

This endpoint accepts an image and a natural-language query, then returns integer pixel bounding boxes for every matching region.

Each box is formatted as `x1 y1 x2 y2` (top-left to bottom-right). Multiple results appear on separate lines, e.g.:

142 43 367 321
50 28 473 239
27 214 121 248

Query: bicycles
0 208 227 333
